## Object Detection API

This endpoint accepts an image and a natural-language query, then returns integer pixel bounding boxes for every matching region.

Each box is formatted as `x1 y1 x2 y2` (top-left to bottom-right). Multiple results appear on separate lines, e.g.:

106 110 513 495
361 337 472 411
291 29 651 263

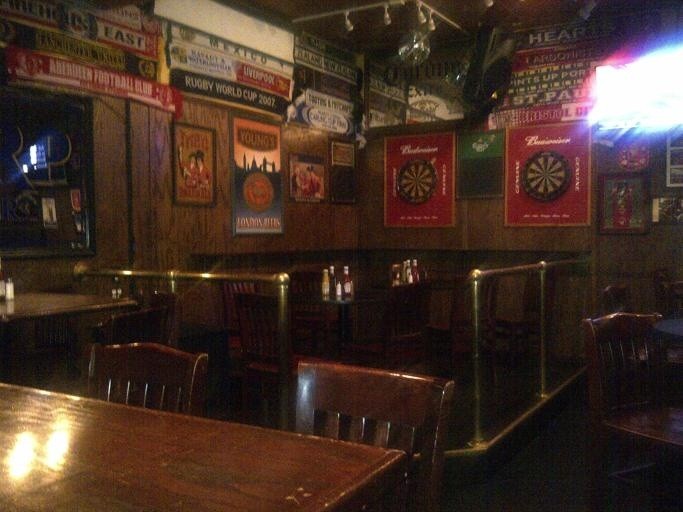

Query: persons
178 146 198 195
196 151 210 198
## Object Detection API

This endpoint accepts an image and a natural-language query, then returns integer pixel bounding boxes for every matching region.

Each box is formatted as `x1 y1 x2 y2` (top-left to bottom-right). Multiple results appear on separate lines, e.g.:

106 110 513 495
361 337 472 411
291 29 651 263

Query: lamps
344 0 437 34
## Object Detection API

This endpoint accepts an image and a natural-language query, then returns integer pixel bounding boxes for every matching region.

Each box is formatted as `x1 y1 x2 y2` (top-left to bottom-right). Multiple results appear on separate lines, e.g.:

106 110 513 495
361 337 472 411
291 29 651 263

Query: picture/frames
600 175 649 234
228 109 285 239
328 135 358 206
173 123 218 208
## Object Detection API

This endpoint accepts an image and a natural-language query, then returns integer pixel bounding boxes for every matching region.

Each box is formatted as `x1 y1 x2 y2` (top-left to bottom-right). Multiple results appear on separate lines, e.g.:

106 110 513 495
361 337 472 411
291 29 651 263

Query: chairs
222 266 496 436
85 342 208 419
573 270 683 512
295 360 454 512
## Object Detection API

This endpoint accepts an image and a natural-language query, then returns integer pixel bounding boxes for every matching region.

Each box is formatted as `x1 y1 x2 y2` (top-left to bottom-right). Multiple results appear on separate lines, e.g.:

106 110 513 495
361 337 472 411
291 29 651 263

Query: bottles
390 259 420 287
0 271 15 301
321 262 353 300
